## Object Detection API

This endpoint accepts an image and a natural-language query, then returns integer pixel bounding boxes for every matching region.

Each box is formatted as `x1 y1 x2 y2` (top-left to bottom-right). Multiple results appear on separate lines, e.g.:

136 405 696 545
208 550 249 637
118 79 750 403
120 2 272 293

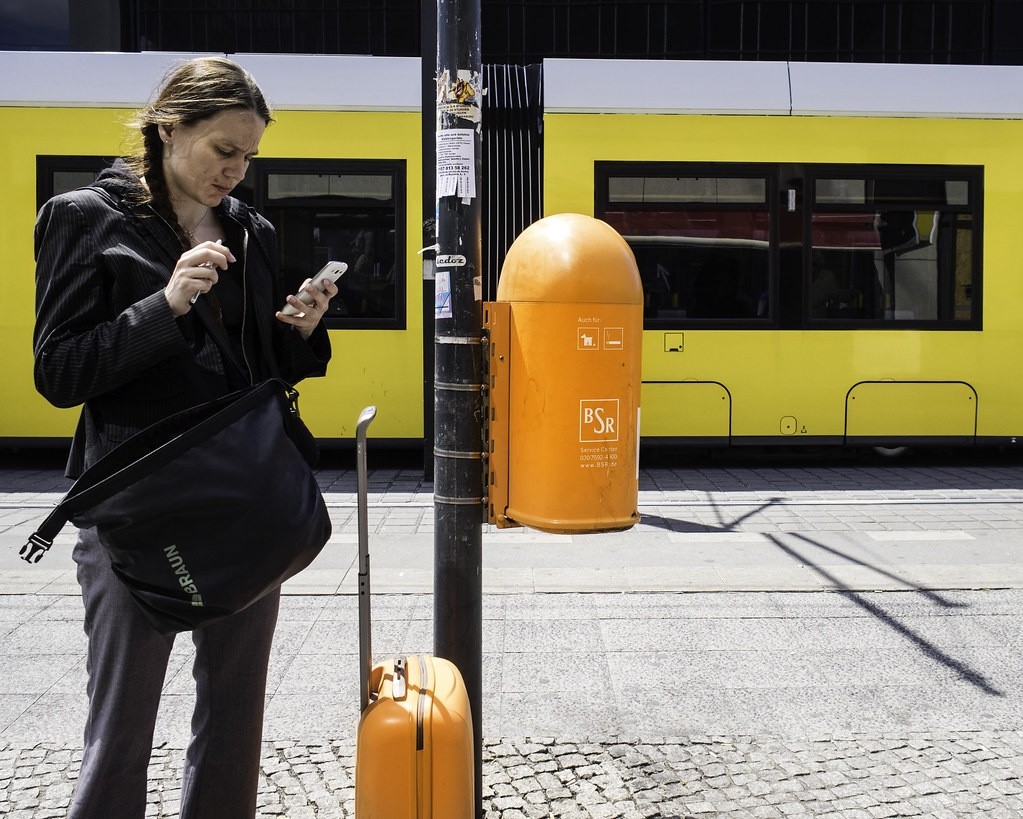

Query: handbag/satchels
17 376 334 633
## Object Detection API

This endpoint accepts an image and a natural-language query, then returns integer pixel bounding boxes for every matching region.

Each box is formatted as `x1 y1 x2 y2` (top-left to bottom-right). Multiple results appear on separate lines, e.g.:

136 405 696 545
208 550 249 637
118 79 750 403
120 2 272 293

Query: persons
16 57 338 819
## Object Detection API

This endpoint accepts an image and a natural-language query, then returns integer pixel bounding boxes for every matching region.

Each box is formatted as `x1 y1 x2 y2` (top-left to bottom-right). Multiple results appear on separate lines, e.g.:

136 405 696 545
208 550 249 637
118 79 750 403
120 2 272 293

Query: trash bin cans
474 205 651 538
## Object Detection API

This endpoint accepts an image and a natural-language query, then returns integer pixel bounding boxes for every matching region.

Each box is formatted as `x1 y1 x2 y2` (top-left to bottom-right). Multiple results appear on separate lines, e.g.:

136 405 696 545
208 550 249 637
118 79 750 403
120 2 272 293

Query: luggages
354 407 475 818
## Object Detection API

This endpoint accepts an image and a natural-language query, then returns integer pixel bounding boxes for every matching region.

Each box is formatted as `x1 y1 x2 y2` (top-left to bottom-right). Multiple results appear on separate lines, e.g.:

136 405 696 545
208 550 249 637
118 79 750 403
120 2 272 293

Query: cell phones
282 261 348 316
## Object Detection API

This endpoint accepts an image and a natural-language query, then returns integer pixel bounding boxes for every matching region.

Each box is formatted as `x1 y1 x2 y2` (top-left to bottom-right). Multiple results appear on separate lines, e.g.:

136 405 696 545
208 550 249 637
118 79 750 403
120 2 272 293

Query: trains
0 47 1022 459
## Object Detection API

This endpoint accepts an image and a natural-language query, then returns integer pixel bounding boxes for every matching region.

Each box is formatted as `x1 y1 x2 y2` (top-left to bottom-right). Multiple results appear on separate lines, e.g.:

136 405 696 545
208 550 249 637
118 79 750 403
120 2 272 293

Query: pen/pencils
188 237 223 306
